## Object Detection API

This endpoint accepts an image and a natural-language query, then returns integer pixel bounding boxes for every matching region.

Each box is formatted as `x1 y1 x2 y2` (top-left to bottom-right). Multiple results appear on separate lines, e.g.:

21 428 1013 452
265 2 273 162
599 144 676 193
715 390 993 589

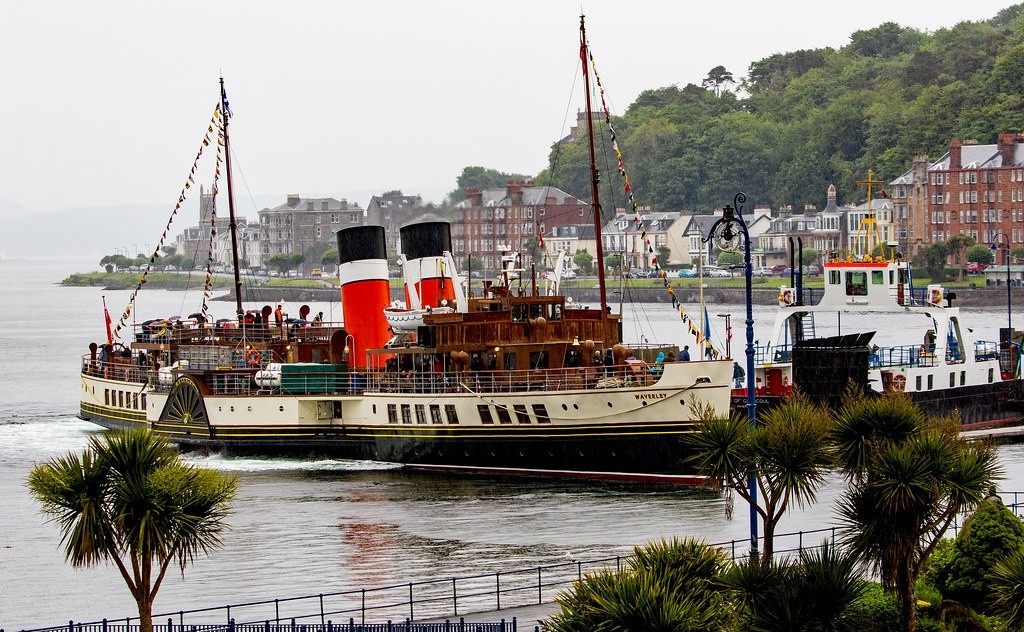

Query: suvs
389 270 401 278
965 263 984 276
311 268 321 278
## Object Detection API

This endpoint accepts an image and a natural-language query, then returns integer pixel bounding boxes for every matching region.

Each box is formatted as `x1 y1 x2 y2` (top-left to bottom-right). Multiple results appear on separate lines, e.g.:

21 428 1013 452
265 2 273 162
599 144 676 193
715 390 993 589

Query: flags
538 232 545 249
106 89 234 349
578 30 732 359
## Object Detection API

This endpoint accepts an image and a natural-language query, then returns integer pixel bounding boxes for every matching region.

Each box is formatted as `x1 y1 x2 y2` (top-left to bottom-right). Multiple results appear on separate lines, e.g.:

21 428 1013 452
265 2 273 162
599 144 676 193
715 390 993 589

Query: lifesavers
784 290 795 304
927 289 942 303
246 350 260 365
893 374 906 390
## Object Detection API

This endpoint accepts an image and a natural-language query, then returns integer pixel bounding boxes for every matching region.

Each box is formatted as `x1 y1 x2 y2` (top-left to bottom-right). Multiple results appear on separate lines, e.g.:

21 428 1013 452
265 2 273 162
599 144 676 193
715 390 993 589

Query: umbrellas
149 313 202 326
283 318 297 323
251 309 262 314
291 319 308 322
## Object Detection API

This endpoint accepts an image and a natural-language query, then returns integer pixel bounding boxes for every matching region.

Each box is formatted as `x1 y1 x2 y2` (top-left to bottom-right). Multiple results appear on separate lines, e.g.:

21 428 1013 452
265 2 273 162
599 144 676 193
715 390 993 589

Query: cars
541 270 577 279
467 271 483 280
621 263 825 279
195 265 302 278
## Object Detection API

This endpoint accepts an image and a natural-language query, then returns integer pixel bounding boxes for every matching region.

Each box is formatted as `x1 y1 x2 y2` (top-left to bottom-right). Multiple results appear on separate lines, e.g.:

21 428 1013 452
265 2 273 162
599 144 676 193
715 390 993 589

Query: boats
73 10 737 494
707 168 1024 430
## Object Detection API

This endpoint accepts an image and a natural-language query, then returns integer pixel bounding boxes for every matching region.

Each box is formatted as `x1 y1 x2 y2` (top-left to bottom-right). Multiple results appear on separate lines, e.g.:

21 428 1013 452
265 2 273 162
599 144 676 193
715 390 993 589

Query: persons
629 346 745 388
244 306 323 342
150 317 226 342
379 341 614 393
